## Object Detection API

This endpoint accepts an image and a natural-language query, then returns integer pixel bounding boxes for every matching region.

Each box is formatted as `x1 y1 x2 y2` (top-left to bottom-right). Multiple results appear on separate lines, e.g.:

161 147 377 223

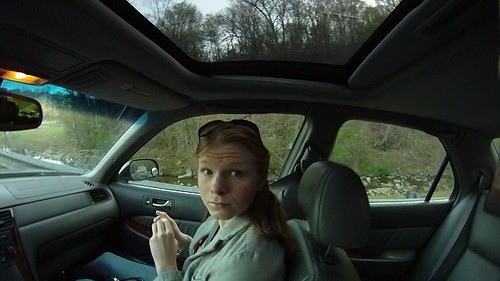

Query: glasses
198 119 264 146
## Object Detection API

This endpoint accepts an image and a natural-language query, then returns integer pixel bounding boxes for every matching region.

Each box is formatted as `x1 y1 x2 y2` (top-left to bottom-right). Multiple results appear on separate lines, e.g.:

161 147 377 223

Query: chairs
402 165 500 281
284 160 372 281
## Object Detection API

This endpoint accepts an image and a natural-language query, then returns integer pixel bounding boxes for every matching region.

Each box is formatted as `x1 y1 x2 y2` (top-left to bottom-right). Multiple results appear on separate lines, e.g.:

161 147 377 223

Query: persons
76 119 294 281
